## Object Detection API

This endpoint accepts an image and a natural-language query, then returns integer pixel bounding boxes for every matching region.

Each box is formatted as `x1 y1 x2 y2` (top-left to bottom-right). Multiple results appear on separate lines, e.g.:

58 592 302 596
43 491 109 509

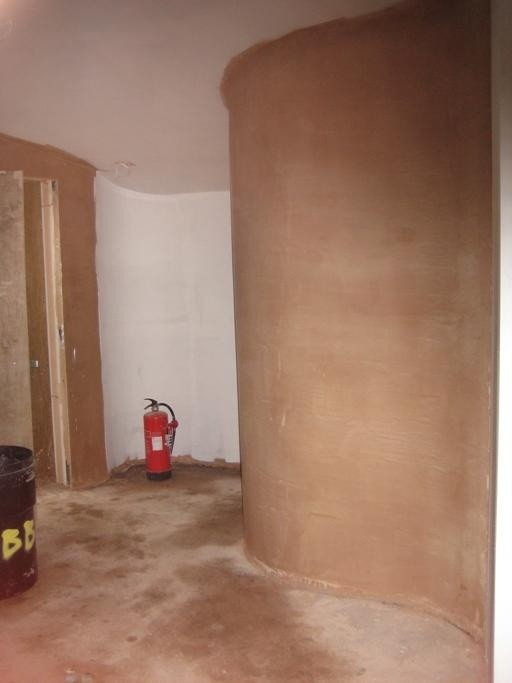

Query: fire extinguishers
144 398 179 481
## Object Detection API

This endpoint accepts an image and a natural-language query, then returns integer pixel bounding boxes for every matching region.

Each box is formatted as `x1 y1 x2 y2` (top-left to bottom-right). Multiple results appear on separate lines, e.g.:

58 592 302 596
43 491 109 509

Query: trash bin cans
0 445 38 599
